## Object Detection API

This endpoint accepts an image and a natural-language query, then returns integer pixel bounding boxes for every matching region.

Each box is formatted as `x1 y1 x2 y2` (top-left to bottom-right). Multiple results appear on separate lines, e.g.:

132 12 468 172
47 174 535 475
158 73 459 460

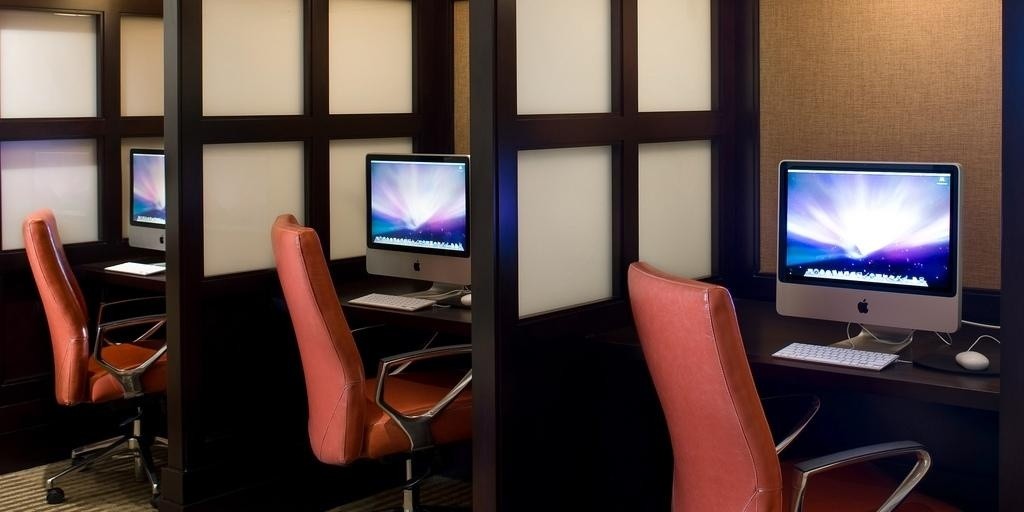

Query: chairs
621 257 962 510
21 209 167 510
272 212 472 512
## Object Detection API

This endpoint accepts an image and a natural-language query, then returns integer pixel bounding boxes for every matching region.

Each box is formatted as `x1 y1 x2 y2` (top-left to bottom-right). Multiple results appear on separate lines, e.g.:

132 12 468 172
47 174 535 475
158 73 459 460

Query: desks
631 295 1003 411
336 283 471 328
69 255 166 294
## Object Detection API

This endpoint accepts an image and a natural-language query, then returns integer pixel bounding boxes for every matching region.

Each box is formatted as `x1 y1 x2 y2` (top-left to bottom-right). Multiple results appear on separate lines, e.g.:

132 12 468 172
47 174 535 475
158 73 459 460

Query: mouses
954 351 990 371
461 294 473 306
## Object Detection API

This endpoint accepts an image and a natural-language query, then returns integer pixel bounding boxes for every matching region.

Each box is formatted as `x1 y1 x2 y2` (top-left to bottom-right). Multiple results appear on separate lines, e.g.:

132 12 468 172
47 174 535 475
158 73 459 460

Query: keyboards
348 292 437 312
770 342 900 372
103 261 167 276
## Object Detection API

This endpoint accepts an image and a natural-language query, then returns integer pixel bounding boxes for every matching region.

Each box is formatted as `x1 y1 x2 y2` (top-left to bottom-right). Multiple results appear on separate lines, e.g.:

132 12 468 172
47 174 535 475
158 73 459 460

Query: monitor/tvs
127 147 166 266
775 159 966 355
365 153 472 300
